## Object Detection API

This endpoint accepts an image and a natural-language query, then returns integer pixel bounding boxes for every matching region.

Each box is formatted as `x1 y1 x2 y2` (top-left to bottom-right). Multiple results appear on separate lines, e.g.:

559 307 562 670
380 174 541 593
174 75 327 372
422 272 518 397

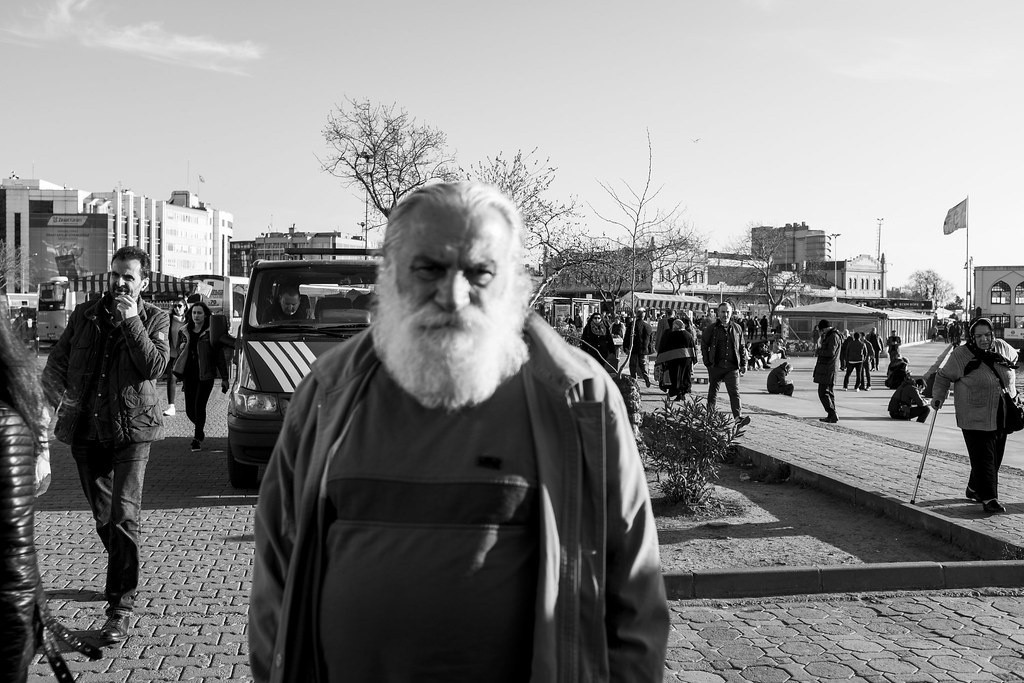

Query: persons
247 181 670 682
931 317 1019 512
0 287 48 683
931 319 969 344
812 319 841 424
732 315 794 397
163 291 202 416
839 326 884 392
260 282 307 324
40 246 170 643
565 306 698 401
694 302 751 430
172 301 229 452
885 331 930 423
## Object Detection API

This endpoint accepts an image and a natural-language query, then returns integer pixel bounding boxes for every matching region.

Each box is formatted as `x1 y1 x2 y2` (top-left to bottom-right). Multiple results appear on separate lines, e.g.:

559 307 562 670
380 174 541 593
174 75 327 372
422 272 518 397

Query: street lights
831 234 840 302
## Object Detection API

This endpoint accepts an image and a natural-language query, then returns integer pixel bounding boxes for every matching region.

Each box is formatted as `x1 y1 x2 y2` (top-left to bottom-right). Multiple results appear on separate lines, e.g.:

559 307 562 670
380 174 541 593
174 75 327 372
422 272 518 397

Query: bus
183 276 249 341
36 277 86 343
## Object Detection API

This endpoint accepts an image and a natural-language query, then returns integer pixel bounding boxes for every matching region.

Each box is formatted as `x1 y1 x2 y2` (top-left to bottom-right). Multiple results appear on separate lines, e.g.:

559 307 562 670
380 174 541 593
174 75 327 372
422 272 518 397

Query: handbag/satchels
659 378 667 392
54 406 82 444
1003 392 1024 431
898 402 910 418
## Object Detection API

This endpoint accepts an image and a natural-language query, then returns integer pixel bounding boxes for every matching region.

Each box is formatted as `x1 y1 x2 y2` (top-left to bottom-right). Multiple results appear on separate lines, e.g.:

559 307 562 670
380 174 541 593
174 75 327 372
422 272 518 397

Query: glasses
593 317 601 319
173 304 185 308
821 328 827 332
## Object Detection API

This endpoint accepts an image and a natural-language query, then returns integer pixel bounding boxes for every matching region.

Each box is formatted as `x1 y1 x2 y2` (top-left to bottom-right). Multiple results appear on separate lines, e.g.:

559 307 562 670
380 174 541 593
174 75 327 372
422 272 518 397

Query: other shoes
764 364 770 368
190 439 201 452
966 487 982 502
200 430 204 442
759 366 765 371
171 351 183 381
982 498 1006 513
752 368 758 371
841 387 847 391
644 375 650 388
854 386 871 392
164 405 176 416
735 415 751 428
819 415 838 423
767 361 771 364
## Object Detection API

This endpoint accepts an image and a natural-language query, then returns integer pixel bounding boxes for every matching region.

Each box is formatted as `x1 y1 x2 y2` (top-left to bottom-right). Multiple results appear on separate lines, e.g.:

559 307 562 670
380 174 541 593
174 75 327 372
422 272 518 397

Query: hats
818 320 831 330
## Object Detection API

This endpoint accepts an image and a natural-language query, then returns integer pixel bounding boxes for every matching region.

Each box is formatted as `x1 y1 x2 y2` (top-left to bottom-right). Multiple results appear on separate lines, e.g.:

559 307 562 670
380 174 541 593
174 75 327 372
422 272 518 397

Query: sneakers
97 614 128 642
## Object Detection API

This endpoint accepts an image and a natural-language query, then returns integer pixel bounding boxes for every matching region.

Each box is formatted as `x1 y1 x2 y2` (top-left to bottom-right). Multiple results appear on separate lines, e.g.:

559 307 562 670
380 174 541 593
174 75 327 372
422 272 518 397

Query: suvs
226 259 380 488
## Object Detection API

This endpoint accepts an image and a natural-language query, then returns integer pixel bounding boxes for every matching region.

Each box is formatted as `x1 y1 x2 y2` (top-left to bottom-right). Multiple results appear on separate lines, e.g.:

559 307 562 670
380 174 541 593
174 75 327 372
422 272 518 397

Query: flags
194 280 212 298
943 198 966 235
